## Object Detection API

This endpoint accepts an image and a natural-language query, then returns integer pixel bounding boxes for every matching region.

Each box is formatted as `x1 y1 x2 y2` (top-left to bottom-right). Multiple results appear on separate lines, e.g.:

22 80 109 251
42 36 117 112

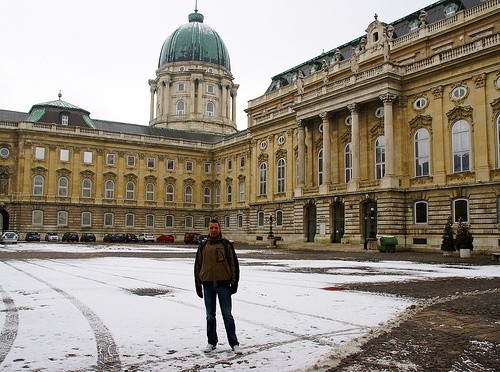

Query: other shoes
232 345 242 354
204 344 217 352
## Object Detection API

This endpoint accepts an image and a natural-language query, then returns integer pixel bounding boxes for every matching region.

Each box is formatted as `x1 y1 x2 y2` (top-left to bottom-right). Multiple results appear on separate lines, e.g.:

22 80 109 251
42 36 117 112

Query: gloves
196 285 203 298
230 284 238 295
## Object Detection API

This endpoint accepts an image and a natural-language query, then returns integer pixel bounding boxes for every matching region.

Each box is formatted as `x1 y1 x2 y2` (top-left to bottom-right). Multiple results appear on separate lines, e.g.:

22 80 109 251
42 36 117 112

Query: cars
80 232 97 242
103 233 122 243
0 231 18 244
193 234 209 244
137 233 155 243
26 232 41 242
121 233 138 243
156 234 175 243
45 231 60 242
61 231 79 243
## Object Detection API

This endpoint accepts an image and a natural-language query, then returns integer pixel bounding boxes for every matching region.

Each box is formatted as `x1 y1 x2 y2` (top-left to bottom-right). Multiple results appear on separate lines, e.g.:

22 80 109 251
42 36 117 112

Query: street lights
266 213 277 249
365 207 379 249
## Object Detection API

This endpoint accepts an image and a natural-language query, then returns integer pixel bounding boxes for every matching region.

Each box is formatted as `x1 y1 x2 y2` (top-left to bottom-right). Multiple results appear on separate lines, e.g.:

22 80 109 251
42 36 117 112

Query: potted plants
455 215 474 258
440 217 456 256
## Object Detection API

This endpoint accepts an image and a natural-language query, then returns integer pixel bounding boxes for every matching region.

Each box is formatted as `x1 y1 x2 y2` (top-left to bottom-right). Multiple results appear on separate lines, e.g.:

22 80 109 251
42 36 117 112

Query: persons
194 220 243 355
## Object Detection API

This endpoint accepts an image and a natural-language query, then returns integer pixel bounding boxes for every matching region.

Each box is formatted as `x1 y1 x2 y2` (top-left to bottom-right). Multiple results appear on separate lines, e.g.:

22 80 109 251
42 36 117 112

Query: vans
183 232 202 245
